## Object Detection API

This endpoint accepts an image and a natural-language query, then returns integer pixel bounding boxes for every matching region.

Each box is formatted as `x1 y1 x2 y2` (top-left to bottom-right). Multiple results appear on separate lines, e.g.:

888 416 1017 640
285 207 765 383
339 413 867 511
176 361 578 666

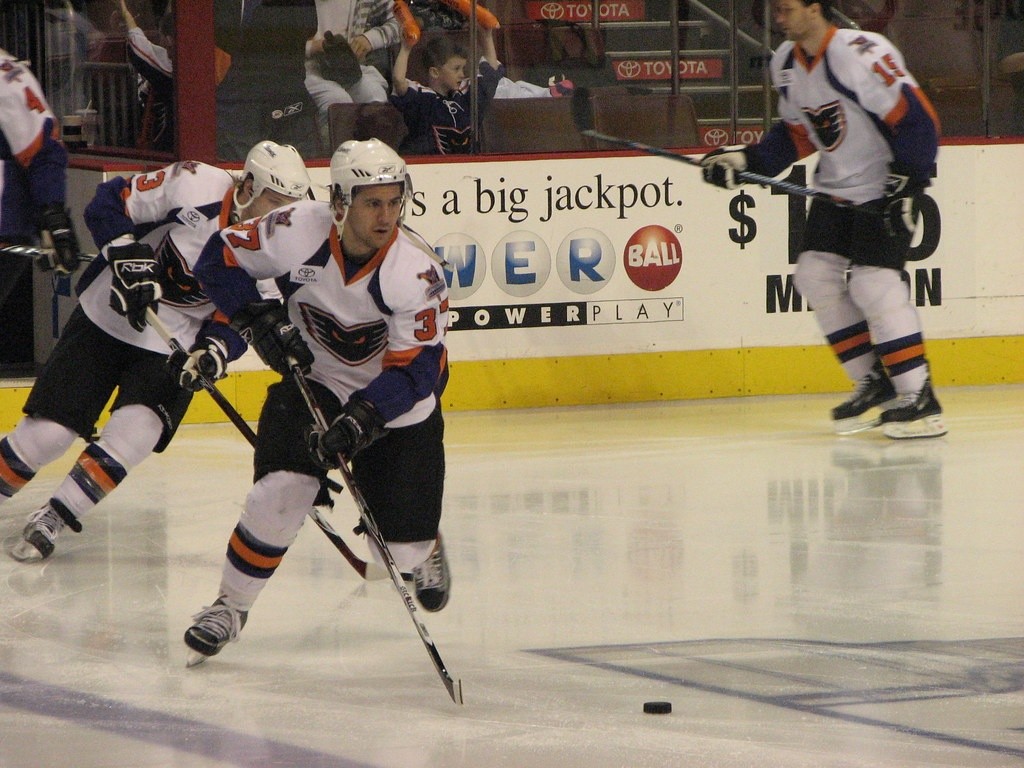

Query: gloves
303 398 390 470
701 148 750 190
35 213 80 278
228 298 315 379
165 319 248 391
107 242 166 333
880 162 931 240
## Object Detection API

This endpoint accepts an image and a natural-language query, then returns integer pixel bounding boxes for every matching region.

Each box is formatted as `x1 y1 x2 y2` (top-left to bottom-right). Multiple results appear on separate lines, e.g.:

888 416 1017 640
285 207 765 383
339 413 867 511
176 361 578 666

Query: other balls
643 701 672 714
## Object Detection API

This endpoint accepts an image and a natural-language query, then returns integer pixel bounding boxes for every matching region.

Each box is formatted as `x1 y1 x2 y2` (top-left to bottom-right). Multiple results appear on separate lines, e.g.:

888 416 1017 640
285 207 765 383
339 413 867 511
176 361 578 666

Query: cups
62 116 81 148
75 110 97 145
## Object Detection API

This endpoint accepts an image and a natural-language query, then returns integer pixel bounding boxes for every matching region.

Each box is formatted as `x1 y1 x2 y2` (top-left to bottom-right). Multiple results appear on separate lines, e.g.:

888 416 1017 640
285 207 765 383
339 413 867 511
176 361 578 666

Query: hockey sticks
573 86 883 217
143 306 391 582
289 352 466 705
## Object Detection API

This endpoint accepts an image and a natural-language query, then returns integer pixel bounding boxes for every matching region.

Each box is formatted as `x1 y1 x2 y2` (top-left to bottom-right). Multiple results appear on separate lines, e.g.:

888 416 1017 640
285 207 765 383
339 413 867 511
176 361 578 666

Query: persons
185 137 451 656
0 48 83 377
0 140 310 560
699 0 949 439
109 0 505 160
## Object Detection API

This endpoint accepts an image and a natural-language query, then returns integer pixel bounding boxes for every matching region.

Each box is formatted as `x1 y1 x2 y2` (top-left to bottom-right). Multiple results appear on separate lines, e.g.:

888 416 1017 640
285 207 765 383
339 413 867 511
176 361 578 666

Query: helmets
241 140 311 197
330 137 407 206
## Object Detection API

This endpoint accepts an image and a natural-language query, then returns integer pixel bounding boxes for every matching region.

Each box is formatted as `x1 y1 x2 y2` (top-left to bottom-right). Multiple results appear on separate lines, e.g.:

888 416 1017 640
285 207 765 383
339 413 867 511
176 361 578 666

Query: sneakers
881 378 949 440
184 598 248 667
414 531 450 612
8 504 67 563
833 360 897 435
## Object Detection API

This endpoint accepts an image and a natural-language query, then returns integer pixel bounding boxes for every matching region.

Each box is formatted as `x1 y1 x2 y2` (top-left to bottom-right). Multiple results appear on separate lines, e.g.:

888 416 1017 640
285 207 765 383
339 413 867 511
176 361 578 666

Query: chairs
328 102 408 158
389 27 481 81
506 22 612 88
478 94 585 153
589 91 701 148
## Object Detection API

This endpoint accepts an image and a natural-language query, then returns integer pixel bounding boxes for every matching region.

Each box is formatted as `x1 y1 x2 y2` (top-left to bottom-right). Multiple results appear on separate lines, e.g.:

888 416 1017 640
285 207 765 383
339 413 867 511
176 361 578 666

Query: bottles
442 0 500 30
393 0 420 43
